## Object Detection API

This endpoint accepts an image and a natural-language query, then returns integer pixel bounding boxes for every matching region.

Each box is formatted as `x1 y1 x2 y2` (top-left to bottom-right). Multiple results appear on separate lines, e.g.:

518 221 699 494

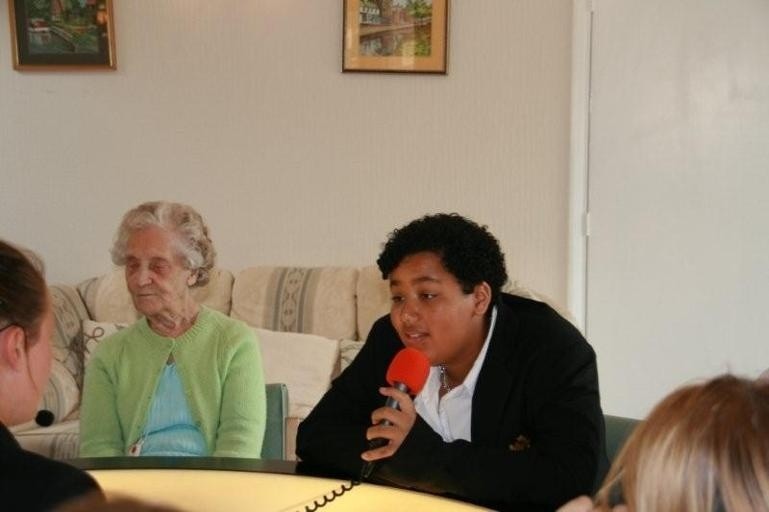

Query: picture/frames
342 0 451 75
8 1 118 72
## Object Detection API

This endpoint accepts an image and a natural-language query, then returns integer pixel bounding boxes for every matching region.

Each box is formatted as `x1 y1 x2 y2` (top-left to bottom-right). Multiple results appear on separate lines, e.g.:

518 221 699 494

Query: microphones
367 346 430 459
35 410 55 427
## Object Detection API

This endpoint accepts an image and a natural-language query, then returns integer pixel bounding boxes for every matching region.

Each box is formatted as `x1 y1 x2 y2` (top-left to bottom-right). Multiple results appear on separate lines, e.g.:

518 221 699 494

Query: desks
66 459 509 512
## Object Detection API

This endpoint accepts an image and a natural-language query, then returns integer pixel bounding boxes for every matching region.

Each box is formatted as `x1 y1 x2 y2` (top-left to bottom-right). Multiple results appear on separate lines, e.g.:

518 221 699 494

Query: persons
556 368 768 512
295 215 618 511
1 241 108 512
78 201 268 461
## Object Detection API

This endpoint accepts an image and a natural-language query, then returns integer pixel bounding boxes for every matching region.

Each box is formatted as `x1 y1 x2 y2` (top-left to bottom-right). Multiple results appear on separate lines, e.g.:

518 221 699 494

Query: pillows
80 317 126 361
77 270 233 324
339 338 365 370
251 328 339 421
236 267 356 344
357 269 393 337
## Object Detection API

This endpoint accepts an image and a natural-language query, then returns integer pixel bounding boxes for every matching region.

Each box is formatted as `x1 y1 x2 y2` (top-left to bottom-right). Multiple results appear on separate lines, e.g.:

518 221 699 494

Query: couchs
9 267 578 469
